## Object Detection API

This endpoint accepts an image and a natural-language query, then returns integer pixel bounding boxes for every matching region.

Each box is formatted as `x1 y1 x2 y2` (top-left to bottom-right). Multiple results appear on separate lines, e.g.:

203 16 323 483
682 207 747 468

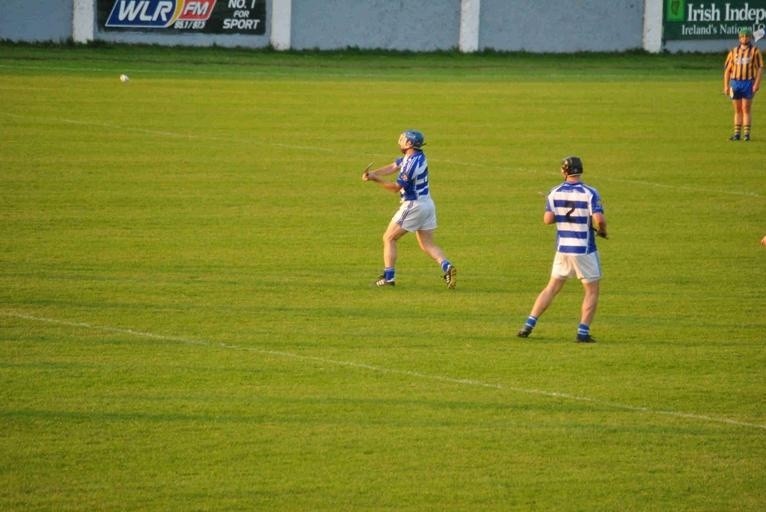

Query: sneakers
518 329 532 338
576 334 596 343
729 134 751 141
444 264 457 290
375 278 395 288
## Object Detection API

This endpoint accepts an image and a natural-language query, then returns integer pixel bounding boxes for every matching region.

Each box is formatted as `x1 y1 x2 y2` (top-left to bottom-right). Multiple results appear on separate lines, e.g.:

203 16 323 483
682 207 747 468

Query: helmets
398 130 424 148
560 156 583 176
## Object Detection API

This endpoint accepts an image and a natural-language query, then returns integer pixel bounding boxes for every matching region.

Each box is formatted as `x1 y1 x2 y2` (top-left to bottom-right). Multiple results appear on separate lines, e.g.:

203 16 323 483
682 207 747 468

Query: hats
738 28 753 37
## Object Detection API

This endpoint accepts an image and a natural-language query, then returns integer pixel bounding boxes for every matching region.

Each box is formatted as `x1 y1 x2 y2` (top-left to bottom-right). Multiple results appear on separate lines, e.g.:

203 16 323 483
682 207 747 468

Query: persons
724 29 764 141
362 129 457 290
516 156 609 343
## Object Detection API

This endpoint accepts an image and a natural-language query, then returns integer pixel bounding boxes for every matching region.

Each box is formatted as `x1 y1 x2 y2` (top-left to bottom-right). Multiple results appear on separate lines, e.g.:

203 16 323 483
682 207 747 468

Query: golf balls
120 74 127 81
